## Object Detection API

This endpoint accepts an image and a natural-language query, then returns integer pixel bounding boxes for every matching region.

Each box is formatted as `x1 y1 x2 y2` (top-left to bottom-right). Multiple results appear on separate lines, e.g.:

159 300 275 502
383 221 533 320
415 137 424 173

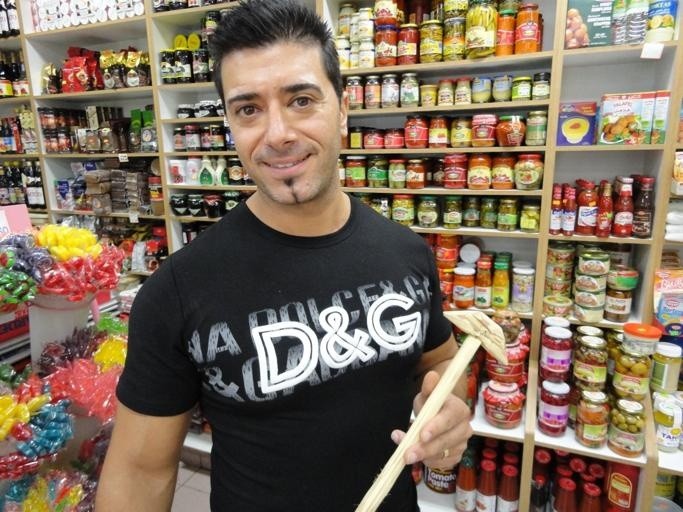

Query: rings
440 447 452 459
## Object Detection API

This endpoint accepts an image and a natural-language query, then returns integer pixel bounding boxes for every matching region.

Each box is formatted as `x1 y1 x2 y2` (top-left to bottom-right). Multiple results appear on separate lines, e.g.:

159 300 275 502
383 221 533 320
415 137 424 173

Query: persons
96 2 474 512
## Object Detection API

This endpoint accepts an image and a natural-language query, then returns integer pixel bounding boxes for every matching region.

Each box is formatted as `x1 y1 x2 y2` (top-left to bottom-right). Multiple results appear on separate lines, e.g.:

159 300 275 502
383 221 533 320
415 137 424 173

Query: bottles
453 436 640 512
197 154 228 187
0 0 20 39
0 50 28 98
450 237 536 313
548 172 654 239
0 116 24 154
0 158 45 210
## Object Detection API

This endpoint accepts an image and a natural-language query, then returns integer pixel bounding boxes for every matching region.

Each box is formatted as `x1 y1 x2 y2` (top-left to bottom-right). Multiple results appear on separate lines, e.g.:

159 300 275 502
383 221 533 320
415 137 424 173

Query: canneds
39 107 86 155
159 9 255 244
337 0 683 458
